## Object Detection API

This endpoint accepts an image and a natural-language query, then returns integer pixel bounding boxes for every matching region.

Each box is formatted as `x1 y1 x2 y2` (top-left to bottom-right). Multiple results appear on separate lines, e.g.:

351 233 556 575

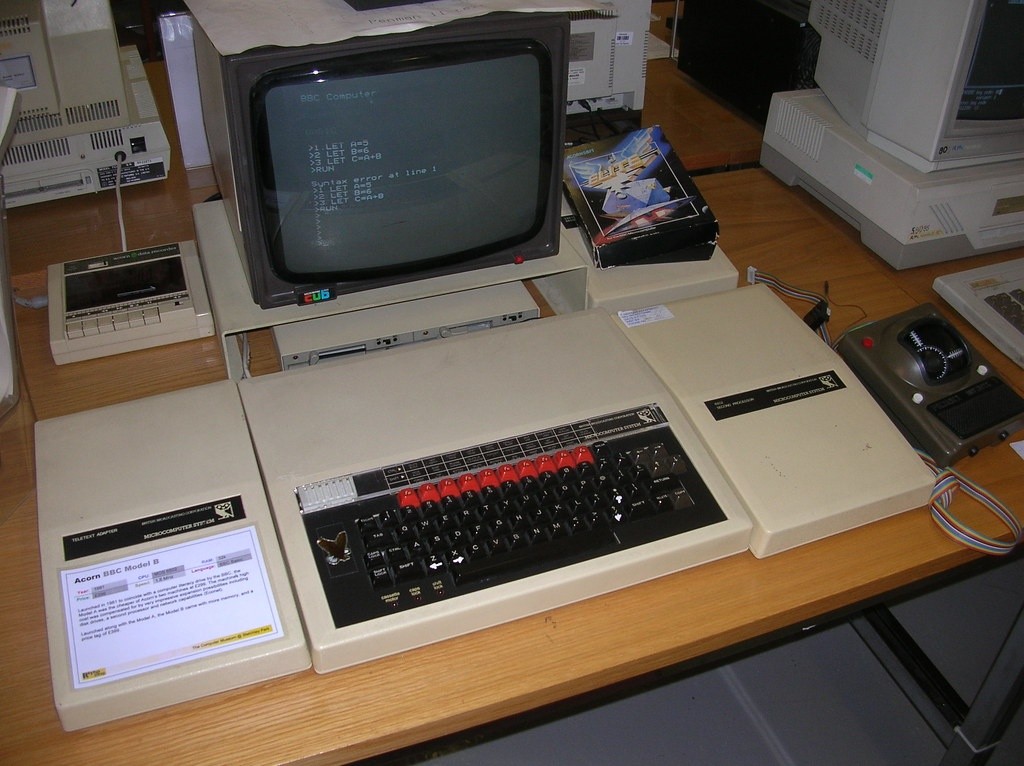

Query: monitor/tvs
0 0 130 149
190 14 571 312
807 0 1024 173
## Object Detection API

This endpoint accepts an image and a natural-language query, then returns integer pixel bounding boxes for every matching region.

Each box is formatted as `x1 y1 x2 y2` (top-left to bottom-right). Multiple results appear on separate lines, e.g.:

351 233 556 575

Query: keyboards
984 288 1024 335
304 425 728 627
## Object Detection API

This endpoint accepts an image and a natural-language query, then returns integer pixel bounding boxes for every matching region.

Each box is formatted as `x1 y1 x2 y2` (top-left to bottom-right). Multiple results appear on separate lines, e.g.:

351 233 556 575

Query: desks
0 0 1024 766
